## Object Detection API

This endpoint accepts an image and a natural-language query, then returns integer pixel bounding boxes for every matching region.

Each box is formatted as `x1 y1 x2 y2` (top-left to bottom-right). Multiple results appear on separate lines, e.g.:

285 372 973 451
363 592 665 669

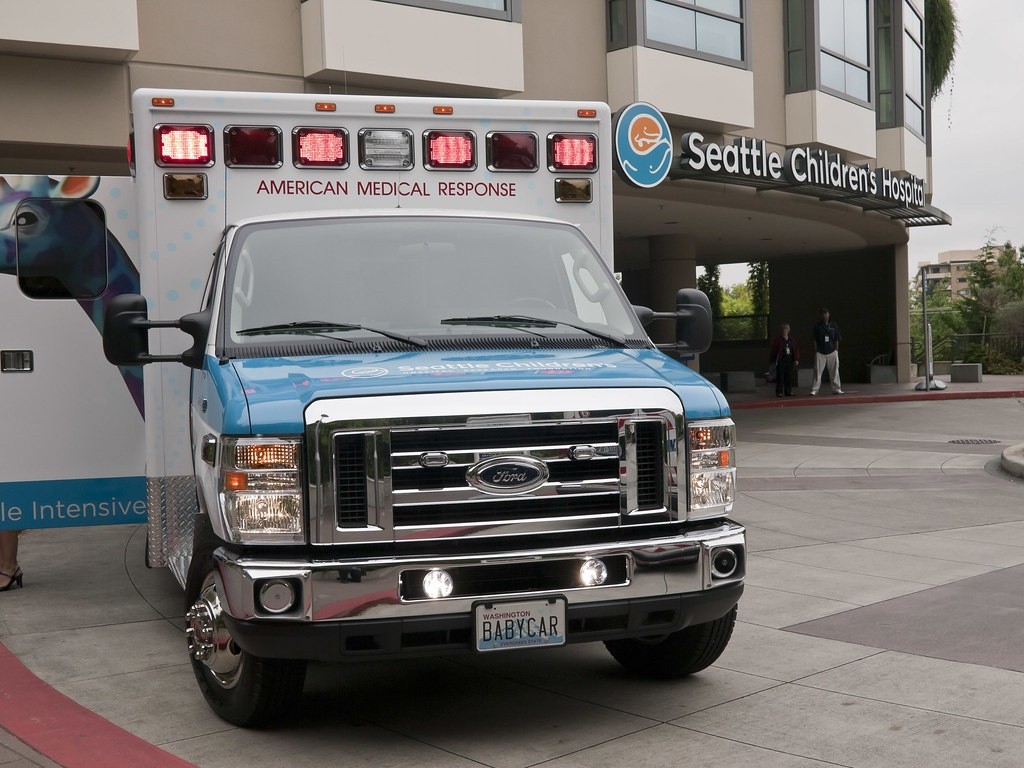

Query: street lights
914 256 999 389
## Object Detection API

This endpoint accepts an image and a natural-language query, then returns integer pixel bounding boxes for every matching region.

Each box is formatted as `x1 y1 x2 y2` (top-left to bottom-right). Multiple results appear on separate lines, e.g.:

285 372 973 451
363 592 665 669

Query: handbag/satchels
766 366 776 382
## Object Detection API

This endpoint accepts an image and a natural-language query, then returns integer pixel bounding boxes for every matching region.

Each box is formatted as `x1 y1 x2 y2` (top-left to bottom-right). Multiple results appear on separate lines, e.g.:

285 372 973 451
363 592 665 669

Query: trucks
1 86 750 733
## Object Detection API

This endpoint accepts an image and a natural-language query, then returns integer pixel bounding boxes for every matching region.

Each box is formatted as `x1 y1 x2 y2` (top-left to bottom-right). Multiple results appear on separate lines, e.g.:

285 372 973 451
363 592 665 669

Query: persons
810 307 845 396
767 321 801 397
0 530 23 592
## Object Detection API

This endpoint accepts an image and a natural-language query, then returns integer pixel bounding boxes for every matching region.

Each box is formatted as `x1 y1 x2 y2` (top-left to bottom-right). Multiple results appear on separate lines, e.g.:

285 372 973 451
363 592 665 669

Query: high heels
0 566 23 591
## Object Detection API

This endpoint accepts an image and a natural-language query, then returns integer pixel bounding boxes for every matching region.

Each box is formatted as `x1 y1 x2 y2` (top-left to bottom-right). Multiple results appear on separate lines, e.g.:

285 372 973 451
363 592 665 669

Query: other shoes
789 393 795 396
810 389 817 395
776 393 782 397
832 389 844 394
